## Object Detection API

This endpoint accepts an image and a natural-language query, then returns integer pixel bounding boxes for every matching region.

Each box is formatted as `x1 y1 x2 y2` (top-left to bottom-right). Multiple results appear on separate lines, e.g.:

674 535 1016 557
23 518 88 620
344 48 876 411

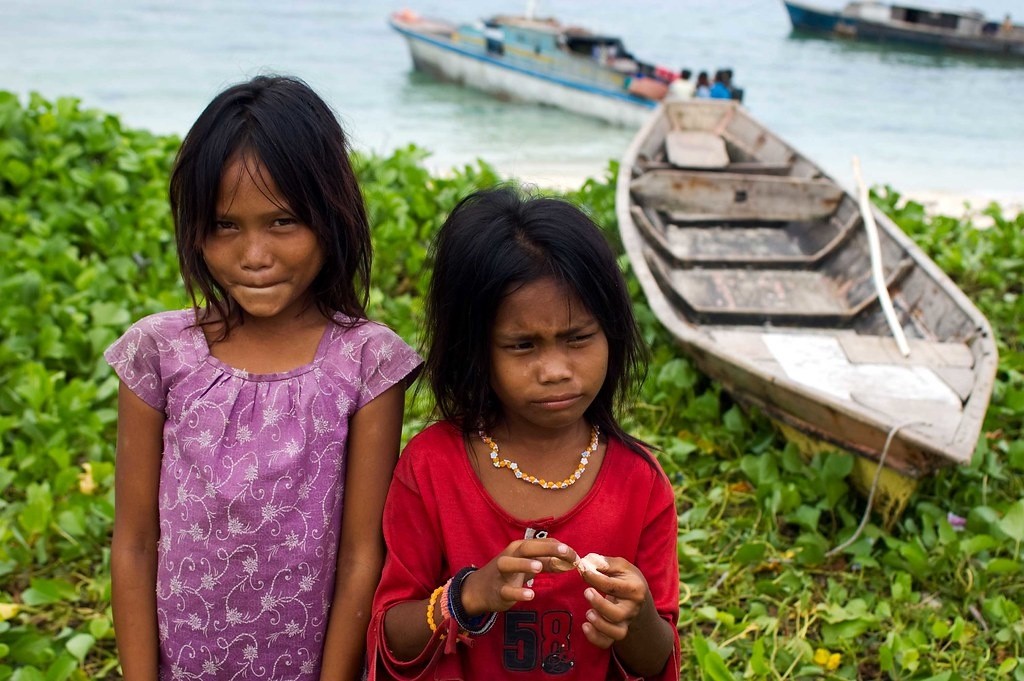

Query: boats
385 0 744 130
782 2 1024 57
615 98 999 537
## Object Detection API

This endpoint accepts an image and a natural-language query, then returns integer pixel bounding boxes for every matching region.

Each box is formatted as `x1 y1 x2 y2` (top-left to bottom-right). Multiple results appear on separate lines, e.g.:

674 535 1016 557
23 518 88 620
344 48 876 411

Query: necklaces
479 423 600 489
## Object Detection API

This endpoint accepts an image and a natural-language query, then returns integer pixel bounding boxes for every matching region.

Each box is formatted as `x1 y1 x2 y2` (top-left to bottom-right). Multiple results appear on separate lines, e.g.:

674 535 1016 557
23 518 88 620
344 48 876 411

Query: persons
668 68 735 100
105 77 425 681
364 188 681 681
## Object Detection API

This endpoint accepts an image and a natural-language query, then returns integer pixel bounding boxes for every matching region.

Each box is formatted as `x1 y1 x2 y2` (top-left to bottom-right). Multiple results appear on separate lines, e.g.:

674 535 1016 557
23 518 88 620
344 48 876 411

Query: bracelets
427 567 498 642
